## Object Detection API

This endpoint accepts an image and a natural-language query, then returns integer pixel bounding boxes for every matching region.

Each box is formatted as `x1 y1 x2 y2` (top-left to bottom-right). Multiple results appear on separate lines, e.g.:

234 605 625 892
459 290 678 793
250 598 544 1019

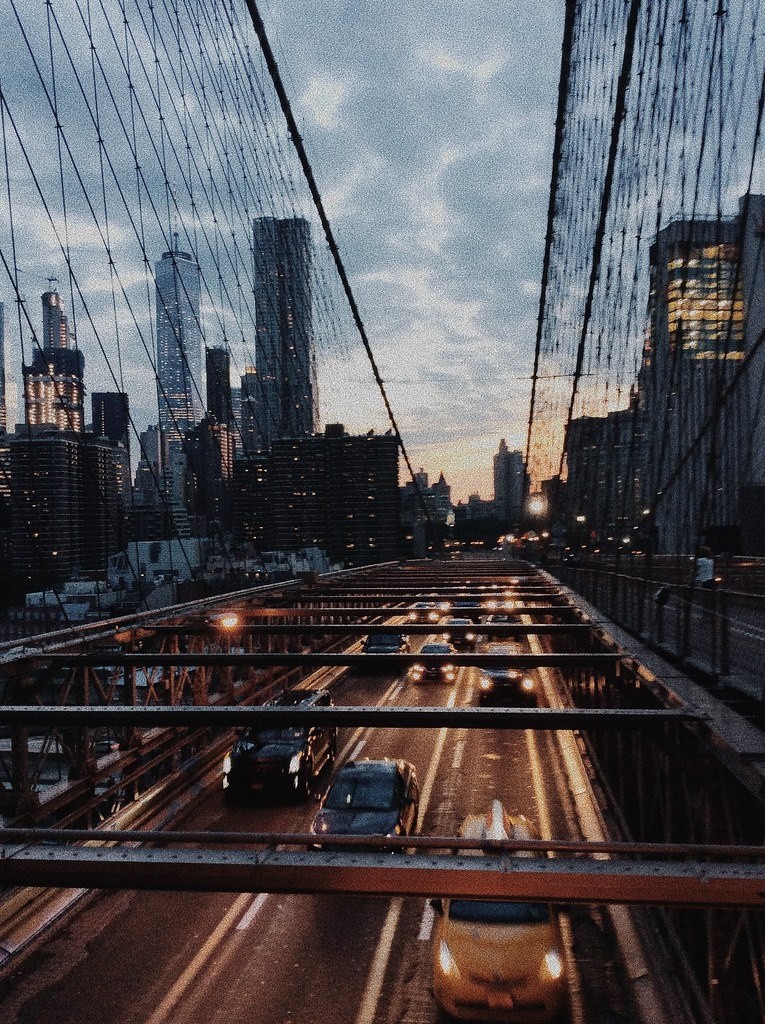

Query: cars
444 618 475 646
430 798 568 1023
478 642 537 700
486 614 523 640
413 643 460 682
305 758 421 856
409 603 439 624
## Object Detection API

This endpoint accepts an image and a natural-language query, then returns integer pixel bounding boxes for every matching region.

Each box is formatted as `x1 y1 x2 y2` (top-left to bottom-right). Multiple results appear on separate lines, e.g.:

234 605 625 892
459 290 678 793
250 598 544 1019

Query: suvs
358 635 410 653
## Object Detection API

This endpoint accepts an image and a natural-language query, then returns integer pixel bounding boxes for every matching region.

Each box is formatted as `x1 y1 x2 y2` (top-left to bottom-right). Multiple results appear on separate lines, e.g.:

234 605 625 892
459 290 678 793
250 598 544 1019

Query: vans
223 688 338 799
